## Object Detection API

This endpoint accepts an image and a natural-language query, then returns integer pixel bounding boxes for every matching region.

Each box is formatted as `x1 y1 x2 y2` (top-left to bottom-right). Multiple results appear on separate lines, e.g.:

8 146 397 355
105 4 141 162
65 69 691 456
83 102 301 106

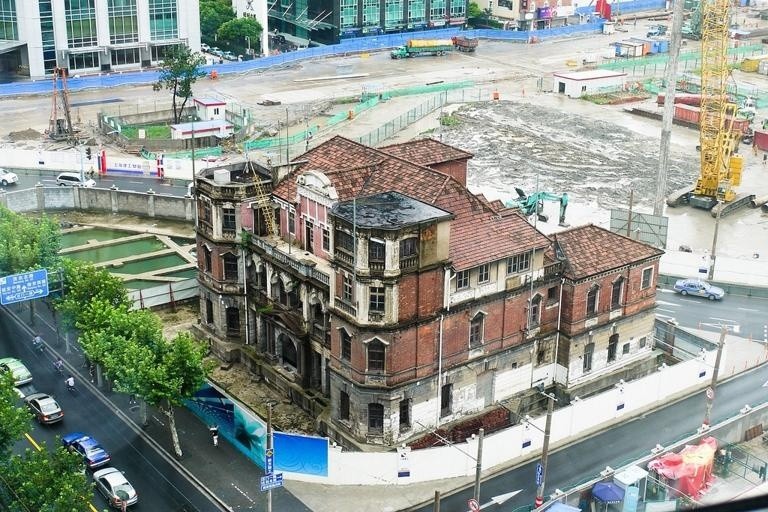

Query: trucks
450 35 479 53
390 43 454 59
646 24 668 38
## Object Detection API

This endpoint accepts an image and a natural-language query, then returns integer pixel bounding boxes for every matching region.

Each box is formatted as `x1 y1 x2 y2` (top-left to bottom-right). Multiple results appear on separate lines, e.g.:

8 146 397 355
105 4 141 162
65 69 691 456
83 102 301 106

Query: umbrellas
115 490 129 501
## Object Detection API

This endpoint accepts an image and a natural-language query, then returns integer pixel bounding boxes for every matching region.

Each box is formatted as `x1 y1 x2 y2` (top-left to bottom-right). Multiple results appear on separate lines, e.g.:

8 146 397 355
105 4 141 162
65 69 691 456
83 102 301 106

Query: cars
23 391 65 426
0 168 19 186
91 466 138 510
0 356 35 387
200 42 238 62
673 278 726 301
61 430 112 470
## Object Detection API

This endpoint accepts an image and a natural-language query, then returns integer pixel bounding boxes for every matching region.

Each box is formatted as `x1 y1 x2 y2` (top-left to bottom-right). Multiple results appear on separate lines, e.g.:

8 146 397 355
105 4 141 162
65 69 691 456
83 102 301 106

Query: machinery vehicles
503 186 573 229
663 0 760 220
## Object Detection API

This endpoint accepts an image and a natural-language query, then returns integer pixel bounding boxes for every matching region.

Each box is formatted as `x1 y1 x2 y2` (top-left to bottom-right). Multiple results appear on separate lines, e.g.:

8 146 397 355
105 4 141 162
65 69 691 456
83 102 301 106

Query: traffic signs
1 270 50 304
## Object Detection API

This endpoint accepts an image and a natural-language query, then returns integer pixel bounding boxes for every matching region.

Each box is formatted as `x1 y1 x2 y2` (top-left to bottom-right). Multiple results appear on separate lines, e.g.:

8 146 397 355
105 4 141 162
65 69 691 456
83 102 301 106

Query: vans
55 172 97 188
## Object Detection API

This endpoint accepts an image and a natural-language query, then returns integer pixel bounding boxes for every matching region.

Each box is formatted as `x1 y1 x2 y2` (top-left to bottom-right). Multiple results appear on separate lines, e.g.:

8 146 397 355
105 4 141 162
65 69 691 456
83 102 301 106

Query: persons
89 362 96 382
129 394 137 405
121 499 127 512
32 333 41 345
62 374 75 386
53 357 65 371
209 421 220 448
